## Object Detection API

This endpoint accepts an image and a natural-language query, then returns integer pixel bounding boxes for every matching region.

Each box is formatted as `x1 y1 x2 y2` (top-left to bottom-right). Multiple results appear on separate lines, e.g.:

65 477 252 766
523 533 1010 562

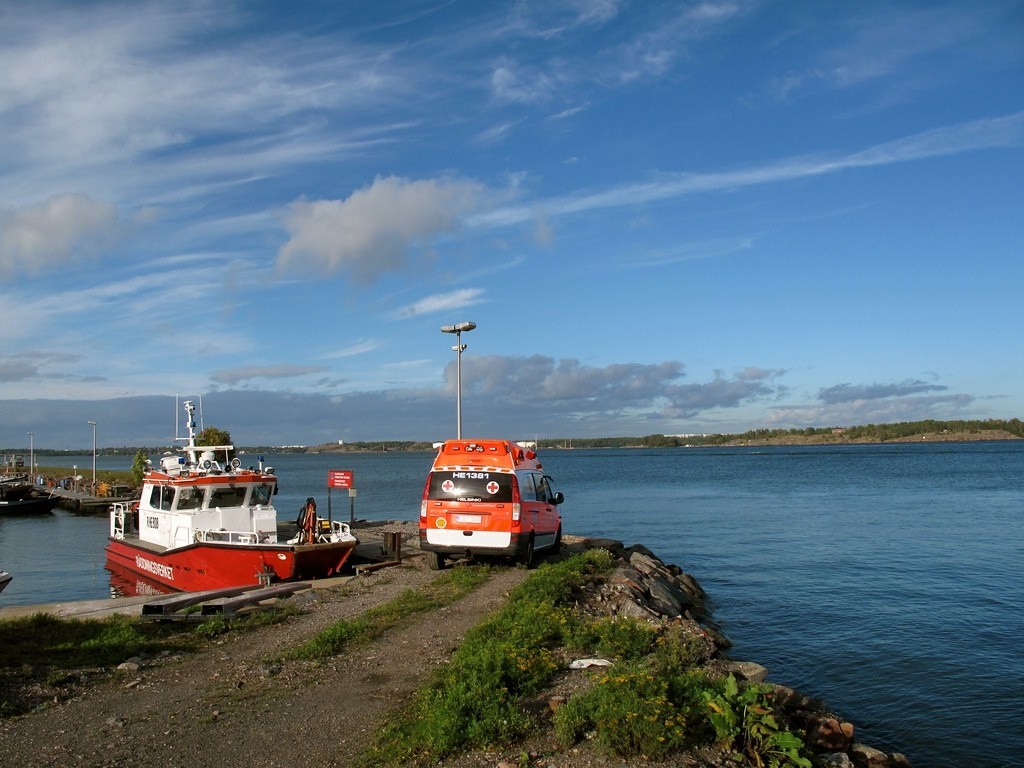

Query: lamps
230 458 242 475
203 459 212 476
265 467 275 475
180 470 190 479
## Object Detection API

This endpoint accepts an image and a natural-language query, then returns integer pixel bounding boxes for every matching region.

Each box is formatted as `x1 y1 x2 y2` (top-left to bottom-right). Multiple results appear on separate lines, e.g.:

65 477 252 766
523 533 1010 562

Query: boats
0 472 62 516
104 392 362 595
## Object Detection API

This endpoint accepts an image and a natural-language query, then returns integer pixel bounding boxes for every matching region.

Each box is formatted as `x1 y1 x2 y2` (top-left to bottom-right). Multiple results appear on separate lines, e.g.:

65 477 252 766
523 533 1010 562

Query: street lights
26 431 34 484
87 420 98 496
440 321 478 440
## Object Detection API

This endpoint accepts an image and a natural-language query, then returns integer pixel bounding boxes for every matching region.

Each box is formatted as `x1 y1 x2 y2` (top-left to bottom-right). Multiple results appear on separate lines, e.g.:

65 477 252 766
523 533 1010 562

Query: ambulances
417 439 565 570
